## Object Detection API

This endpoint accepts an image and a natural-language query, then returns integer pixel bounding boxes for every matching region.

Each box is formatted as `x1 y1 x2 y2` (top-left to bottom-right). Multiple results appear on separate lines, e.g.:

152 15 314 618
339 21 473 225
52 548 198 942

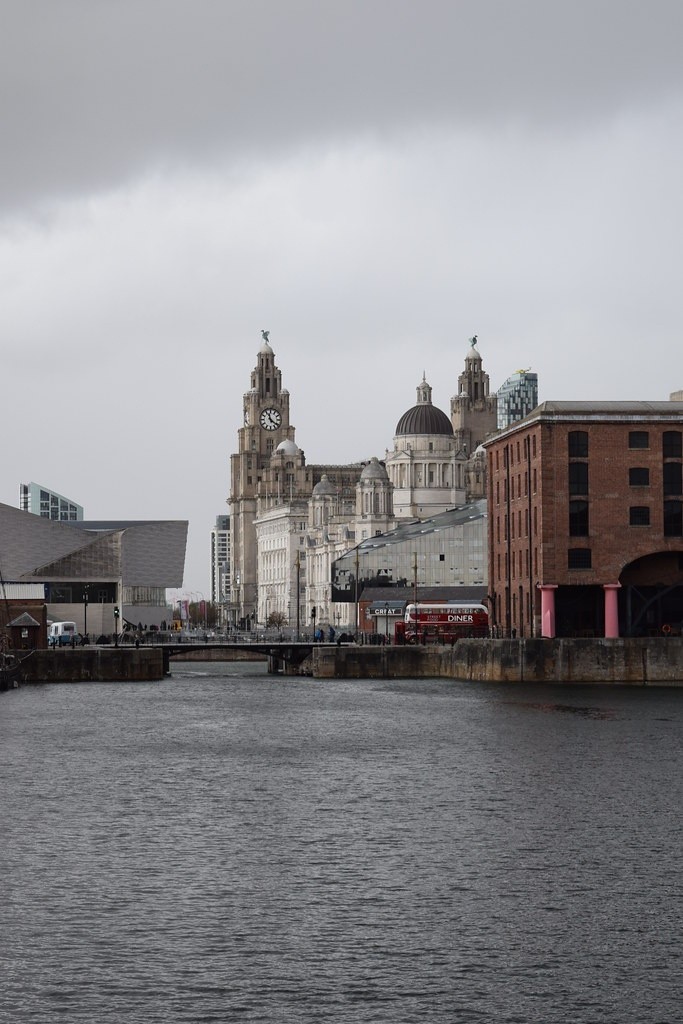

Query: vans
47 622 78 647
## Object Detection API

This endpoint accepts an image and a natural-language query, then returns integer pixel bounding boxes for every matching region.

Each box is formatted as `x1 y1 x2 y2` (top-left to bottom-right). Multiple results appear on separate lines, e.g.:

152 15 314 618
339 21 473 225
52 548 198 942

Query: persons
512 627 517 639
315 627 335 642
431 627 440 645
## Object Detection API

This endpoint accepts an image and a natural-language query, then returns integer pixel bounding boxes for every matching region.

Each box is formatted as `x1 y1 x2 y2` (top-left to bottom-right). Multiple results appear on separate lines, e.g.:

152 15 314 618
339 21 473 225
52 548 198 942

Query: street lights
385 601 390 643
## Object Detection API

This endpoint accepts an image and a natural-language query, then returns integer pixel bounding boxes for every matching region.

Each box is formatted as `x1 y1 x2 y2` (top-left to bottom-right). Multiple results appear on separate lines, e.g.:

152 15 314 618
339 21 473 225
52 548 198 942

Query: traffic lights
311 609 316 617
113 607 119 619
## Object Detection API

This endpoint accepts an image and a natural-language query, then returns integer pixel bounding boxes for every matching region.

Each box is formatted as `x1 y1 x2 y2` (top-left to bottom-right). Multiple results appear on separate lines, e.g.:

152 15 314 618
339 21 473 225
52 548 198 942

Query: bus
395 621 474 646
405 602 491 644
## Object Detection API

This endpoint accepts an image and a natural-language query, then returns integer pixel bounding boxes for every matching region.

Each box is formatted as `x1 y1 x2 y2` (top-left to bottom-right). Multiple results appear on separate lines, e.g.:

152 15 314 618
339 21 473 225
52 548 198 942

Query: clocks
259 408 282 431
